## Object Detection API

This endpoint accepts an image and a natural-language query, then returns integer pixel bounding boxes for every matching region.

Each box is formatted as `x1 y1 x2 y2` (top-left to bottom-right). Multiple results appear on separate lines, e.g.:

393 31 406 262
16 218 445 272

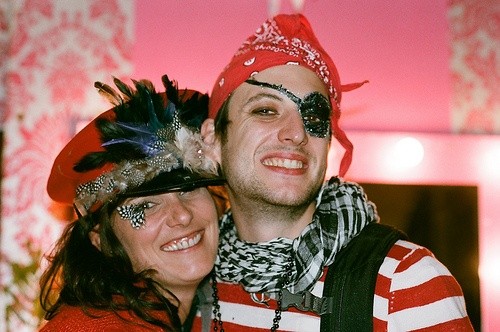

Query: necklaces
212 220 293 332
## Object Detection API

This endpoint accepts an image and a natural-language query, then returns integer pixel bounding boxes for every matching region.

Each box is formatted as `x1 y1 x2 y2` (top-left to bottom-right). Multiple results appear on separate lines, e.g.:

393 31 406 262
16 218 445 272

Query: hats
47 75 229 219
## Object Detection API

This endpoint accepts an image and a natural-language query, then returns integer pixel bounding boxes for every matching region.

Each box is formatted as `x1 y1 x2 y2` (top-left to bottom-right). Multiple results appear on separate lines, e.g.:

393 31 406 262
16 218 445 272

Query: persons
38 73 227 332
190 14 478 332
